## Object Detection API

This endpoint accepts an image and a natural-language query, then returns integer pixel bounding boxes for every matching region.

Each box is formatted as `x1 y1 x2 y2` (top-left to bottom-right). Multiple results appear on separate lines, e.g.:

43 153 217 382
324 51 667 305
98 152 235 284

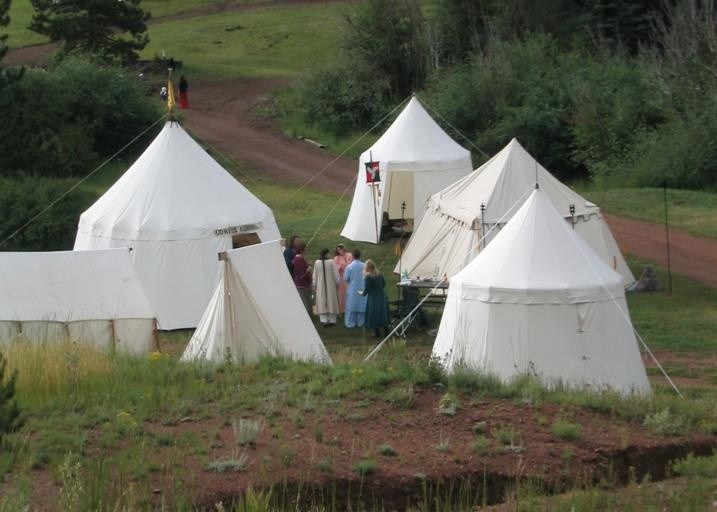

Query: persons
160 86 167 101
357 258 392 338
343 248 368 329
283 236 301 277
294 244 313 317
178 75 188 109
311 248 341 326
334 244 353 314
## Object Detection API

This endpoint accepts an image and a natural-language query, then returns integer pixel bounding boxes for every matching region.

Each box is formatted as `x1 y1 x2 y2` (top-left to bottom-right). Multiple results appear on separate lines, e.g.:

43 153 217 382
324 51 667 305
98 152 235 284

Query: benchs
387 294 447 314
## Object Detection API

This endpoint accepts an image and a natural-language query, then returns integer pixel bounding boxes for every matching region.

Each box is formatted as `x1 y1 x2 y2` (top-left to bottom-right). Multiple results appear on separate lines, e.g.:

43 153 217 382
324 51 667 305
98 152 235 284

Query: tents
179 238 335 366
339 94 473 245
430 183 652 401
72 114 285 332
393 137 638 297
0 250 158 357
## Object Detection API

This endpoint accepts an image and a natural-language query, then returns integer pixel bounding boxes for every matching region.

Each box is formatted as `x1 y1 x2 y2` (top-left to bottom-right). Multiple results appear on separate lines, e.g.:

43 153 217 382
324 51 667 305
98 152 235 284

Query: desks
396 279 449 314
